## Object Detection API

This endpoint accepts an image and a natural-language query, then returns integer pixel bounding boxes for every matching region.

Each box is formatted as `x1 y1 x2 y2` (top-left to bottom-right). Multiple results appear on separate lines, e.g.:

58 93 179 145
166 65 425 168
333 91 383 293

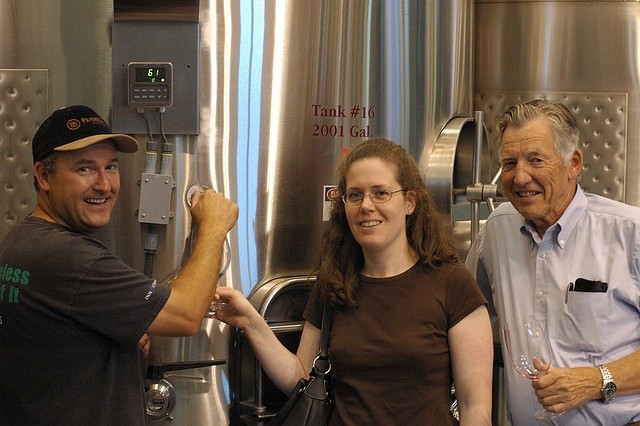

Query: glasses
341 188 406 206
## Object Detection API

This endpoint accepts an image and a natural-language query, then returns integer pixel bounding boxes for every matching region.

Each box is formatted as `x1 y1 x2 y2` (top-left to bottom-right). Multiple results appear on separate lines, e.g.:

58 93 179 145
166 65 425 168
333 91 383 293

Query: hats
32 104 140 164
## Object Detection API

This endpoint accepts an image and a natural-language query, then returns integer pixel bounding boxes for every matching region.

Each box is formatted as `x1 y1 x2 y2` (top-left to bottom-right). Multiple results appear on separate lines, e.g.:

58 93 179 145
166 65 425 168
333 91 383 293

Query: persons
1 99 240 426
206 135 496 426
464 98 640 425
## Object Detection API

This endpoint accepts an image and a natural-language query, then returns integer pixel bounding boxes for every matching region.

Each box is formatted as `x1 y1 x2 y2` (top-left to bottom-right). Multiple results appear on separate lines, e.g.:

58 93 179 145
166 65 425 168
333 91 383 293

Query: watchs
596 364 618 406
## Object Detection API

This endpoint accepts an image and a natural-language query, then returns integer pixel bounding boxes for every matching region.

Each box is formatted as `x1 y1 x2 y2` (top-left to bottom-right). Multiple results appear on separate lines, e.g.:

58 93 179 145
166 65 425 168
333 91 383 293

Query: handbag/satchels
270 304 335 425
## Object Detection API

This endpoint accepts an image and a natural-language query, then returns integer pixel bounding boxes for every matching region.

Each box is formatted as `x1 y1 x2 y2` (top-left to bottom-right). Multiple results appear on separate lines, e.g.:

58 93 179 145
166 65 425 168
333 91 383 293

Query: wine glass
191 223 236 321
502 314 569 420
